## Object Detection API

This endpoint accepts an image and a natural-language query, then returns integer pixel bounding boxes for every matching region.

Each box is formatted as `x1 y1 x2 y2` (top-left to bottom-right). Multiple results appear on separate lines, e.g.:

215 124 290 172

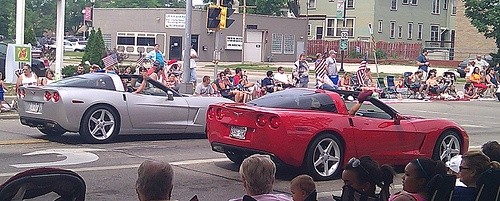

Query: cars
457 56 492 77
45 35 88 52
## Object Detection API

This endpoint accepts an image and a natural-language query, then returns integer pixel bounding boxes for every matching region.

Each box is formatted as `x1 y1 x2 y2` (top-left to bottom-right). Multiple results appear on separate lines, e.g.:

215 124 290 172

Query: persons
418 48 430 79
342 156 394 201
446 141 500 201
195 68 256 103
398 70 440 99
42 69 54 85
136 159 174 201
0 72 8 112
17 64 40 86
182 42 197 80
290 174 316 201
365 68 373 83
73 43 183 94
389 157 447 201
229 154 291 201
464 55 500 99
260 50 350 90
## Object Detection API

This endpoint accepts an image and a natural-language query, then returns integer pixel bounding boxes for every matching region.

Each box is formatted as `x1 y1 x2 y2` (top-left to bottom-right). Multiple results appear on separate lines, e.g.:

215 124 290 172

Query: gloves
358 89 373 103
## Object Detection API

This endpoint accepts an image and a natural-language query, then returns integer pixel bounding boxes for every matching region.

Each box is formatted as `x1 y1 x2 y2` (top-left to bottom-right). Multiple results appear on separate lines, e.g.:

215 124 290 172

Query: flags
101 53 118 67
137 57 144 66
357 52 367 85
315 58 326 78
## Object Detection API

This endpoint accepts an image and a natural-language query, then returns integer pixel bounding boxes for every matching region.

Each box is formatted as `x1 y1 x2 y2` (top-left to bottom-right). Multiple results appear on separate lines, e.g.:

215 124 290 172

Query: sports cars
206 87 469 180
18 70 237 143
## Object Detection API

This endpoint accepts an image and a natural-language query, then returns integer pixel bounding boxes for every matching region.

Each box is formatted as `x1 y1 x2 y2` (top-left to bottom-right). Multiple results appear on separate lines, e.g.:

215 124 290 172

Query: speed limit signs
341 32 348 39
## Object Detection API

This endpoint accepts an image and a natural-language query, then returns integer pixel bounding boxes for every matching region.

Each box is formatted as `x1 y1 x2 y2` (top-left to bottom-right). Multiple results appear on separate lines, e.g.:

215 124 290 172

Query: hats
446 155 462 173
329 50 337 55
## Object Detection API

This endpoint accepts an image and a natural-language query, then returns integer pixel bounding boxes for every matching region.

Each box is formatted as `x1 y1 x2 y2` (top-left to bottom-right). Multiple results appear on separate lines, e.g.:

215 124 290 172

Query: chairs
333 167 500 201
377 71 500 99
299 97 312 106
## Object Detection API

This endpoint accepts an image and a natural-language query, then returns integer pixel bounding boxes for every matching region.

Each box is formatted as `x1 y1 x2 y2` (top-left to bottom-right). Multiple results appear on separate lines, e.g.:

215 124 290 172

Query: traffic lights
207 6 222 29
221 4 236 28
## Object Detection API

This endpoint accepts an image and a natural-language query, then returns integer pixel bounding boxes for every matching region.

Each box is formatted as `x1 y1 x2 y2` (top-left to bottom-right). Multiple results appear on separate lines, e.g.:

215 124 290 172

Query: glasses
458 166 472 172
333 59 335 63
348 157 372 176
